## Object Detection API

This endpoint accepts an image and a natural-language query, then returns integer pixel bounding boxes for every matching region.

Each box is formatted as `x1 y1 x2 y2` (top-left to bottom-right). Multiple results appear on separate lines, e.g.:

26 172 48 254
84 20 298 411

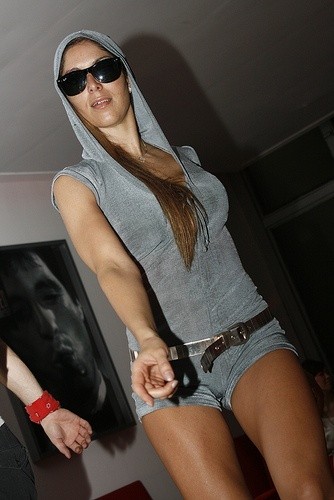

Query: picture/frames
0 239 137 464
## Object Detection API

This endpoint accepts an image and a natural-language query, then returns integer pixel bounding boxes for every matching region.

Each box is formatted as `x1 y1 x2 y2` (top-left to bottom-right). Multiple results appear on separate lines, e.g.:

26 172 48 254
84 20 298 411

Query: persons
50 29 334 499
301 357 334 458
0 248 127 441
0 336 93 500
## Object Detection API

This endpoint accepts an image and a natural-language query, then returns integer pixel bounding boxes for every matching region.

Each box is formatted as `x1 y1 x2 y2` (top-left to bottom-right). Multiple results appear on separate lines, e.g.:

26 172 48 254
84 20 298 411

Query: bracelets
24 390 61 424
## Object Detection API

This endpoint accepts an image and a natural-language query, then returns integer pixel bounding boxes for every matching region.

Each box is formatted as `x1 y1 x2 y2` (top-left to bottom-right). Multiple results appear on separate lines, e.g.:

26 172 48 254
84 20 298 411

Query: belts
128 306 275 373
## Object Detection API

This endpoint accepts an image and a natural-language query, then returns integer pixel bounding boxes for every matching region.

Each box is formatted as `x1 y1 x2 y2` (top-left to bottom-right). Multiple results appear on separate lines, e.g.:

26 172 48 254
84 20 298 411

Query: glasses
57 57 122 96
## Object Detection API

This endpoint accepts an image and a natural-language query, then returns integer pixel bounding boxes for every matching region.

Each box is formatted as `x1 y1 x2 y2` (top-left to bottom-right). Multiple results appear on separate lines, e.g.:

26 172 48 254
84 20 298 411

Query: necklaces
132 135 148 163
